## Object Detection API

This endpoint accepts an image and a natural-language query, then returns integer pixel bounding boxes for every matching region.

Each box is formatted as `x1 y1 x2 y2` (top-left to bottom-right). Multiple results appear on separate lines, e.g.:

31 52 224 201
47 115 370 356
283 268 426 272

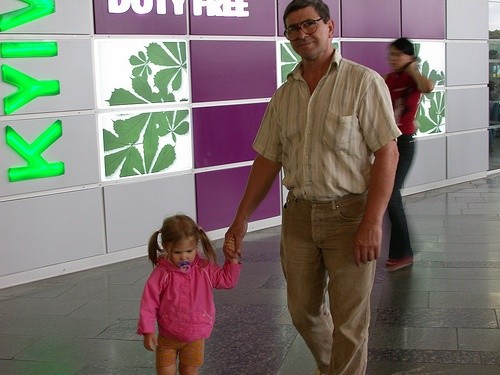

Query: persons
137 215 241 375
223 0 403 375
489 80 500 101
381 37 435 272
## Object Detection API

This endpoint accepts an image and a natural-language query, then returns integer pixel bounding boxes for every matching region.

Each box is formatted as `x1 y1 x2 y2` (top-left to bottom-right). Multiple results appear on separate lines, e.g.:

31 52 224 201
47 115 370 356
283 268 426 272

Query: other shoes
384 258 412 271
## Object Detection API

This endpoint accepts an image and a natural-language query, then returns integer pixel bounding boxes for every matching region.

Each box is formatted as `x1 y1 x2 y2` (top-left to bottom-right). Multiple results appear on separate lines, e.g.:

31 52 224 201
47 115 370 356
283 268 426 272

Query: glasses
284 18 322 40
